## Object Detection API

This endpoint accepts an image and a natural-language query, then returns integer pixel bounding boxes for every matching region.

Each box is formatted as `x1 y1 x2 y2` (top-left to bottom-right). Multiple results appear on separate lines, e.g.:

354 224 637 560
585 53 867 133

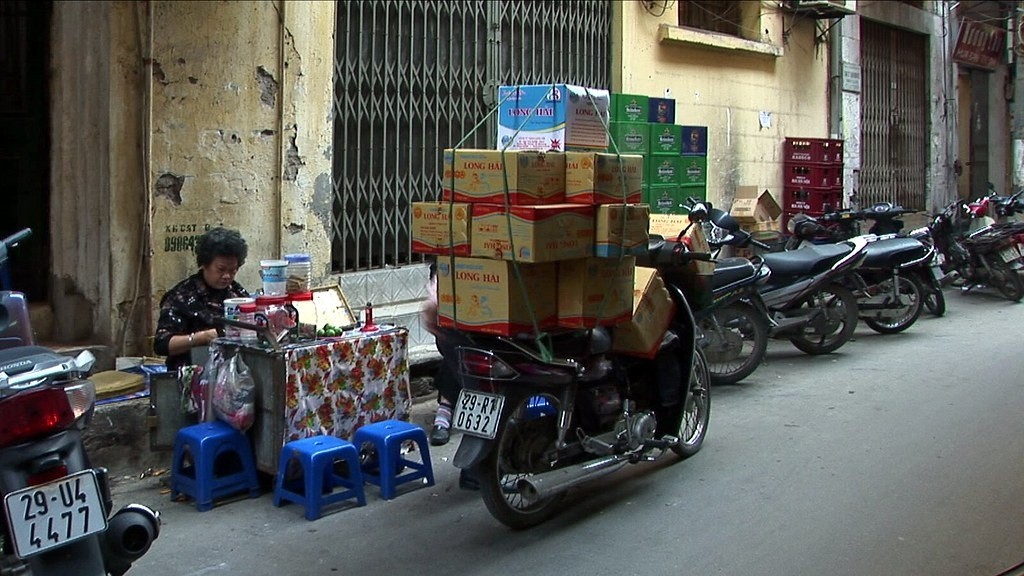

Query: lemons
317 323 342 337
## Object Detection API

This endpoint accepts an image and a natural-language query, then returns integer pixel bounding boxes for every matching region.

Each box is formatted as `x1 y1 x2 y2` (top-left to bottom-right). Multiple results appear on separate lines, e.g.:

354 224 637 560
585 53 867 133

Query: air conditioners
797 0 857 16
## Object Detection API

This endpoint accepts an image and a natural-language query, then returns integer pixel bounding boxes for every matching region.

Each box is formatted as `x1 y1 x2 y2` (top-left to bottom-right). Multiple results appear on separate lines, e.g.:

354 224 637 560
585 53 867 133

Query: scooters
0 222 161 575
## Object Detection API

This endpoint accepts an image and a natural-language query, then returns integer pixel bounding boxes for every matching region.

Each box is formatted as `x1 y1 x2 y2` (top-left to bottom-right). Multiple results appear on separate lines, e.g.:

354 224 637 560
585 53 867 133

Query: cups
260 259 288 296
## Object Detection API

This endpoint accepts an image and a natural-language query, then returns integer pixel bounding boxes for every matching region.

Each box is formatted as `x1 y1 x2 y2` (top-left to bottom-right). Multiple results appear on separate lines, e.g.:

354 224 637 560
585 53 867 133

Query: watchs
187 333 195 347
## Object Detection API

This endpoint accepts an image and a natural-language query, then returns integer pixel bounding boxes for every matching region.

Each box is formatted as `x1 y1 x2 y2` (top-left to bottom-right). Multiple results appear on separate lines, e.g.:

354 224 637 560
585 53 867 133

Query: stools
353 418 435 500
273 435 366 521
170 421 259 513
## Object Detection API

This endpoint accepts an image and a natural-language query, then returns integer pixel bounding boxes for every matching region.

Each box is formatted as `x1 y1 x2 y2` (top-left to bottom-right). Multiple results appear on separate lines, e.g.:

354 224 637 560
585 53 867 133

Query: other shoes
431 420 453 444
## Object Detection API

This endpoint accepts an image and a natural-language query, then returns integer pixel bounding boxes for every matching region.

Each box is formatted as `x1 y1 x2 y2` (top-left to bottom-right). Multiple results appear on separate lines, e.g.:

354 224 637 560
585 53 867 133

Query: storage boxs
409 82 845 361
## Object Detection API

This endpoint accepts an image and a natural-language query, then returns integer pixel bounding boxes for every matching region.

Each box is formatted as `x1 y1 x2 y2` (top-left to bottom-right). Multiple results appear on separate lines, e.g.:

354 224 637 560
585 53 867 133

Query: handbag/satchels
215 352 254 434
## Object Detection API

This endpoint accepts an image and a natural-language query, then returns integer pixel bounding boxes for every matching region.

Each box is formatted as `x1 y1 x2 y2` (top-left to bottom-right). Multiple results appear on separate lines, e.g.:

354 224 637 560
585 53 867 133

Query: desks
209 322 413 479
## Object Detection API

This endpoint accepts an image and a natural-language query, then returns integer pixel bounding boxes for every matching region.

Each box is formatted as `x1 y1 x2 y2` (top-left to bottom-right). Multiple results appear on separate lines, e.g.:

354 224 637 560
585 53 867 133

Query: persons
153 228 250 372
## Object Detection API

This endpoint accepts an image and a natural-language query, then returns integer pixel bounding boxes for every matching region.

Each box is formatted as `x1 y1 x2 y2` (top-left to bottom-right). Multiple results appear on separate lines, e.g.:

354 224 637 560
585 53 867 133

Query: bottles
238 303 258 344
284 253 312 294
792 141 841 199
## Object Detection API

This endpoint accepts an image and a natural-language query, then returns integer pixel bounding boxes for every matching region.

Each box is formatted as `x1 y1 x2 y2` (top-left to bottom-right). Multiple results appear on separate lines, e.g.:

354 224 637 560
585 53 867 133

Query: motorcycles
451 198 712 530
683 179 1024 385
